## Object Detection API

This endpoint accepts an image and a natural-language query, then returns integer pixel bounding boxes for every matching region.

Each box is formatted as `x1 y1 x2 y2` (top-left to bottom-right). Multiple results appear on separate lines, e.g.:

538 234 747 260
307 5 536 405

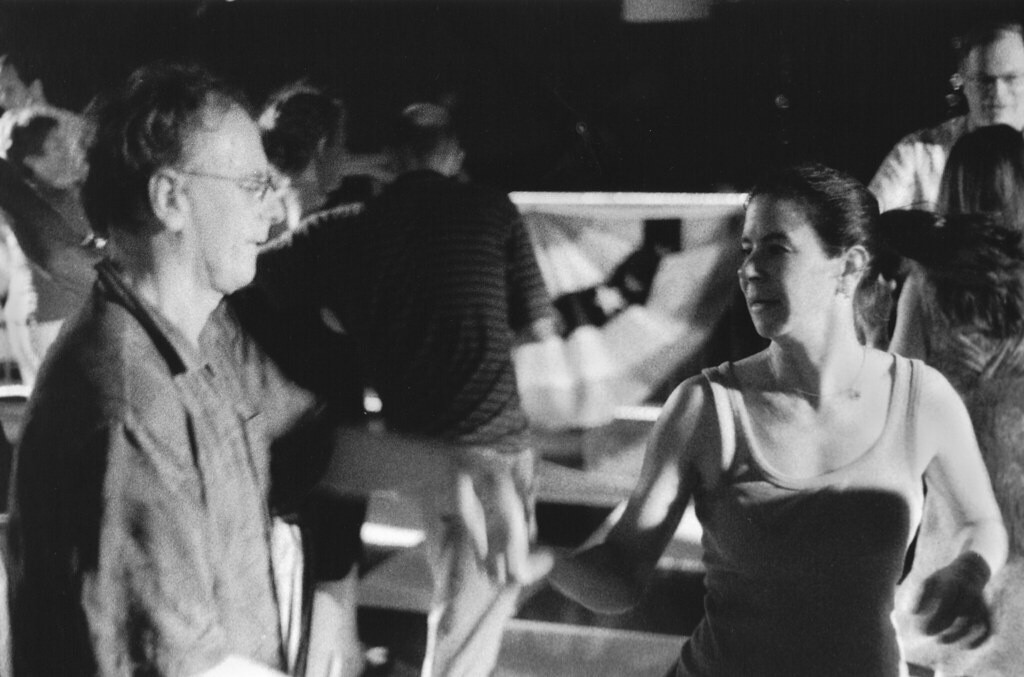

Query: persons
866 16 1024 221
222 76 382 426
1 50 312 676
243 100 553 551
473 154 1012 677
885 123 1024 677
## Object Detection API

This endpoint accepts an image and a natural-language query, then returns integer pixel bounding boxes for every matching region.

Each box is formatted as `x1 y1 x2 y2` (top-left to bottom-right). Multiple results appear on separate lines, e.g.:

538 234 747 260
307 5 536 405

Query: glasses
179 164 278 206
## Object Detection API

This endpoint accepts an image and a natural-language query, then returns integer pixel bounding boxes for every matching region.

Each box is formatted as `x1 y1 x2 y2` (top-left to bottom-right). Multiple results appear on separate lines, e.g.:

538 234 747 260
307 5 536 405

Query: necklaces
765 347 864 397
